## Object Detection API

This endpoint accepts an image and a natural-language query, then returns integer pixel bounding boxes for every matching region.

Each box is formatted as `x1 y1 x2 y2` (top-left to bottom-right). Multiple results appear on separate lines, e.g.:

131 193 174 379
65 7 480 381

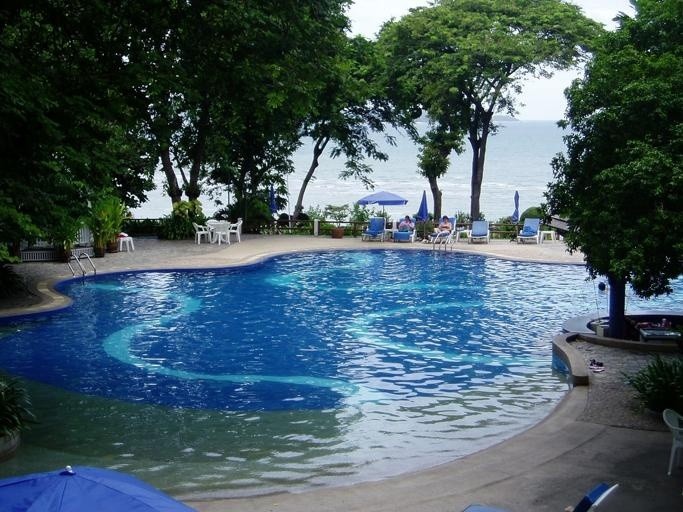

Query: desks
539 230 556 245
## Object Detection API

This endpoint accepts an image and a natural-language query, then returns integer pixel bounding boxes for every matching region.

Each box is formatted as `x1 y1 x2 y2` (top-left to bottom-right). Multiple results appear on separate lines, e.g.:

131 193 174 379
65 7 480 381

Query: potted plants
323 202 350 238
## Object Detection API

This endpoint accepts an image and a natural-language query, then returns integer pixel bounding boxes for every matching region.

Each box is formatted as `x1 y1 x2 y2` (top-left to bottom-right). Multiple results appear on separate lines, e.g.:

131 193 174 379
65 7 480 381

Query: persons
432 215 451 235
397 214 416 232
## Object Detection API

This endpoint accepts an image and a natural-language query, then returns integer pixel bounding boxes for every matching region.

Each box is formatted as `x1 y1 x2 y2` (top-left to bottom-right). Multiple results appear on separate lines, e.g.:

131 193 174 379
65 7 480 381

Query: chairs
360 216 490 245
662 408 682 476
461 482 618 512
516 218 539 244
191 219 242 246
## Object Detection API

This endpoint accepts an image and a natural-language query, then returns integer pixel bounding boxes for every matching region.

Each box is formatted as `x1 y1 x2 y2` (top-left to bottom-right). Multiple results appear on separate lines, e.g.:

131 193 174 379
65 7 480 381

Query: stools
116 236 134 252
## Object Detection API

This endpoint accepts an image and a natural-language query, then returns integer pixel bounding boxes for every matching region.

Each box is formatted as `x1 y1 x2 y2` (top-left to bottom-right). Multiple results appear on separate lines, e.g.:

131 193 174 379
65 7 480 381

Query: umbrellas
512 190 521 225
356 189 408 233
269 183 277 219
0 462 200 511
415 190 428 241
